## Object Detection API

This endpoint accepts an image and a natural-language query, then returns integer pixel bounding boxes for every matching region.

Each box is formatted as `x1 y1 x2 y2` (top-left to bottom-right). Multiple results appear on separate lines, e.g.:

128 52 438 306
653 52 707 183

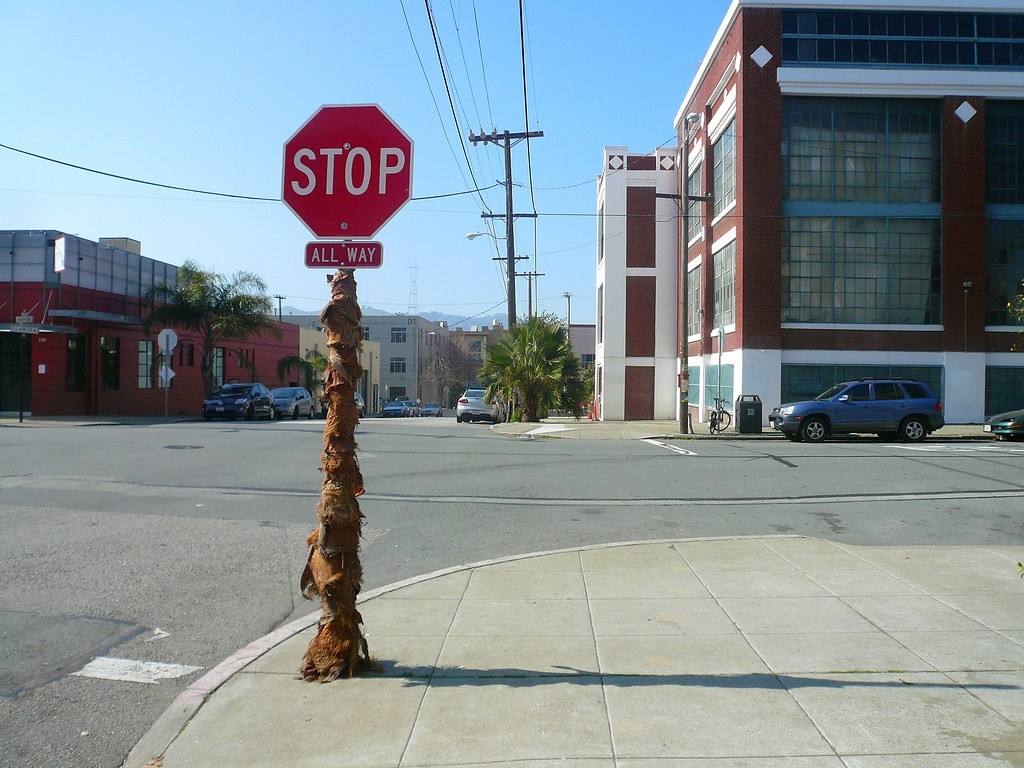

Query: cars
382 401 408 418
323 392 365 419
399 400 429 418
200 383 276 421
269 386 315 421
421 403 443 417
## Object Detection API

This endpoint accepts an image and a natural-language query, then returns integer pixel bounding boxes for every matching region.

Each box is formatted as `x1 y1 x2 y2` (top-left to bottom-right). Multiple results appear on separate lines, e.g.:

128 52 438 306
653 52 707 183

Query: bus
980 408 1023 442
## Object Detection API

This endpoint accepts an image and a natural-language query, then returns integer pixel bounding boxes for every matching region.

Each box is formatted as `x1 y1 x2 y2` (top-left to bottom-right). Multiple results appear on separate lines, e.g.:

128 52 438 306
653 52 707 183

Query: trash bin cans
735 394 762 433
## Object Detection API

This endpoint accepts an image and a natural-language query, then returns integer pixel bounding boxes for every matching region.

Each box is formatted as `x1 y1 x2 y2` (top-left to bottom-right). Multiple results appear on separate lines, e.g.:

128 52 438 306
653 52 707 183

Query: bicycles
708 396 730 435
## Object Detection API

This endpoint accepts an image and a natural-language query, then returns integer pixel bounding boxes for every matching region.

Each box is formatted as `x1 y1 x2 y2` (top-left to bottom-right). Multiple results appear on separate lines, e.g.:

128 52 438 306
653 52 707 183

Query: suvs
457 388 501 424
767 377 945 444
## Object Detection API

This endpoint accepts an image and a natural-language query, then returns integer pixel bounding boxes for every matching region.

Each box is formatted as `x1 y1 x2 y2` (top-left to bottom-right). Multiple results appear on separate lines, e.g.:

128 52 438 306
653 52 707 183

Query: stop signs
281 103 413 240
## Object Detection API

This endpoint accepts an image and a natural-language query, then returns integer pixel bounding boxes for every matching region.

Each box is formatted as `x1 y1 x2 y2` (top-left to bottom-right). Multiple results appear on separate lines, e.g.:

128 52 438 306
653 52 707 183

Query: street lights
466 231 516 330
679 112 699 431
